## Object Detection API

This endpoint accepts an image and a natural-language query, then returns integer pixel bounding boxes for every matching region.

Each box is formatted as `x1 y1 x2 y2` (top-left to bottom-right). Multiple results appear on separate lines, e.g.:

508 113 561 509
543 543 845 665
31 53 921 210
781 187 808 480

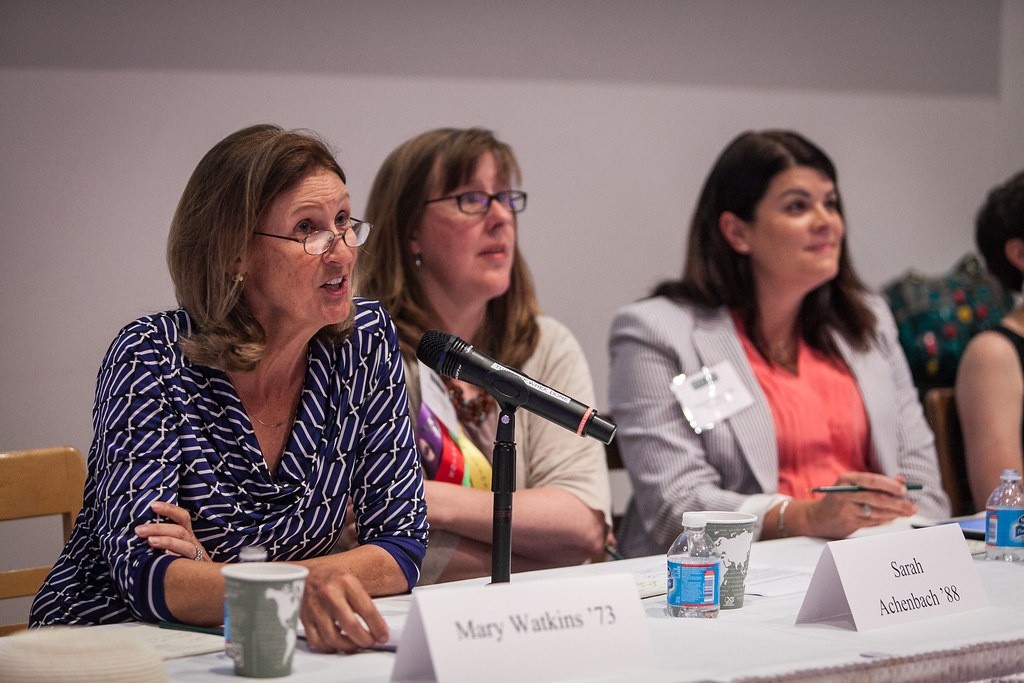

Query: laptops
912 518 986 542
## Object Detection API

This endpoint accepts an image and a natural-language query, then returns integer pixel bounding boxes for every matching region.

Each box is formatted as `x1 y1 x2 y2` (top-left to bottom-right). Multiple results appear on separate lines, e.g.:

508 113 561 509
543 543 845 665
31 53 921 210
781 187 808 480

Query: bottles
665 512 721 619
983 468 1024 566
222 545 269 658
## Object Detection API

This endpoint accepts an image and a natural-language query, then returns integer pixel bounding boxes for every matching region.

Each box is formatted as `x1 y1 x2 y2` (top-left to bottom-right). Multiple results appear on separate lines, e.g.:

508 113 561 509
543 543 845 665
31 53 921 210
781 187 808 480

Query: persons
609 131 950 559
26 125 432 653
947 169 1024 522
336 128 616 588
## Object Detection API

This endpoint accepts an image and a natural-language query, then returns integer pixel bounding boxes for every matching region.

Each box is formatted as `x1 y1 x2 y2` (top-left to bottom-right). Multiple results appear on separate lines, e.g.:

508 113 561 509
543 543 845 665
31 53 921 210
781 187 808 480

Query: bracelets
777 501 790 530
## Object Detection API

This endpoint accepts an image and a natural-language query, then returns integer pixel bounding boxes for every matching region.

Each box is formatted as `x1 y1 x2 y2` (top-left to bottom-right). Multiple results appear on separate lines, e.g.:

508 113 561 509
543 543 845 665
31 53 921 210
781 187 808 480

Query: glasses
253 217 375 256
423 190 528 215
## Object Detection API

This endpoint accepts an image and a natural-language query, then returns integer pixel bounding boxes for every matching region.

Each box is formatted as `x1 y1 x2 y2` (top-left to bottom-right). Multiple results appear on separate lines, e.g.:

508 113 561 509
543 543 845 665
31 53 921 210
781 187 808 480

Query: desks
0 508 1024 683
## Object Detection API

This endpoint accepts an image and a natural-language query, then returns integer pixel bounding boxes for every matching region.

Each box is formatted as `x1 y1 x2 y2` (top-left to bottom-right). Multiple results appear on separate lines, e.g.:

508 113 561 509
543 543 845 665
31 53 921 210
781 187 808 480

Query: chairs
1 445 84 638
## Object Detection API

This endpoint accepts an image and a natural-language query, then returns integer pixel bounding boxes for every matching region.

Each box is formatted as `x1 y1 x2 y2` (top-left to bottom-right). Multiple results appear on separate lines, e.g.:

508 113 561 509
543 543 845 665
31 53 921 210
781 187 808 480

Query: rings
859 504 872 520
194 546 203 561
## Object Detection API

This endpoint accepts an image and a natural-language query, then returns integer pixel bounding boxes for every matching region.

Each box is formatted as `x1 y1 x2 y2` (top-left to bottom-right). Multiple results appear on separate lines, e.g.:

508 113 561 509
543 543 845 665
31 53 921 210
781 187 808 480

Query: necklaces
228 371 293 427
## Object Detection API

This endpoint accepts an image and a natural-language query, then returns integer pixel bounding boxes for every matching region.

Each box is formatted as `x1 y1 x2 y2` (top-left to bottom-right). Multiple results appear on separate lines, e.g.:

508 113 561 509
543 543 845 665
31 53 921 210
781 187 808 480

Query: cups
697 510 759 610
221 561 309 678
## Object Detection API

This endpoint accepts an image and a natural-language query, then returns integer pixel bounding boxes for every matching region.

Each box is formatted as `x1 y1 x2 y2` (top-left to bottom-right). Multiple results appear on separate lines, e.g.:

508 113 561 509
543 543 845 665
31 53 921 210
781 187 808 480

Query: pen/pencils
602 545 625 560
809 482 923 493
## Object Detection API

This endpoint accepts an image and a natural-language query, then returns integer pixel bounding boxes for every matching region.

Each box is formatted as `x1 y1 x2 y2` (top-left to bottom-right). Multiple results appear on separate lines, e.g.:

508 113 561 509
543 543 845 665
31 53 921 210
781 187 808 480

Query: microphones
416 331 618 446
